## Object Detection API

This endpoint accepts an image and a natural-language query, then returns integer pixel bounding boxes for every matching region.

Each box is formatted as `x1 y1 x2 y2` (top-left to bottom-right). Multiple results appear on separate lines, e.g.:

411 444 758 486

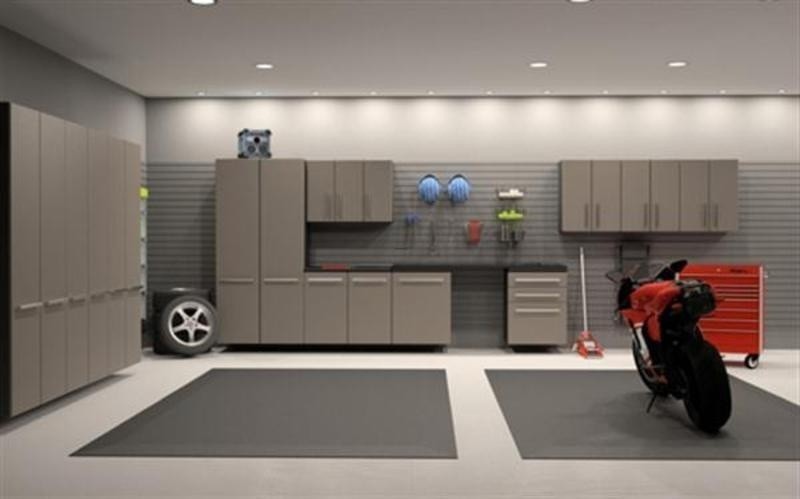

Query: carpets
70 367 459 458
484 368 800 461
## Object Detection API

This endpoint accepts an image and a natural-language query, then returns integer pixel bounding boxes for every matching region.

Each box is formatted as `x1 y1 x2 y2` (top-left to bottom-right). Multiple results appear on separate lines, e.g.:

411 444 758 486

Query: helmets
417 173 442 205
446 174 472 205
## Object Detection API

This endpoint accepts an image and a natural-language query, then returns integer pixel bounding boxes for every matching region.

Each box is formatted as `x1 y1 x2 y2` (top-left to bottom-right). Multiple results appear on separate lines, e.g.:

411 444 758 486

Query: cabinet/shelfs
112 133 141 373
559 161 622 233
4 99 63 416
622 160 680 234
305 270 393 346
218 157 306 345
506 272 567 346
64 118 110 398
681 160 738 234
391 272 450 346
307 159 395 222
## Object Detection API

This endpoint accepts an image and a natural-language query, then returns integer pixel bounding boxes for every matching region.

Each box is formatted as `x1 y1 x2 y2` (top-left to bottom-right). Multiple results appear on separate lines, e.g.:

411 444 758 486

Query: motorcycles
605 259 732 433
